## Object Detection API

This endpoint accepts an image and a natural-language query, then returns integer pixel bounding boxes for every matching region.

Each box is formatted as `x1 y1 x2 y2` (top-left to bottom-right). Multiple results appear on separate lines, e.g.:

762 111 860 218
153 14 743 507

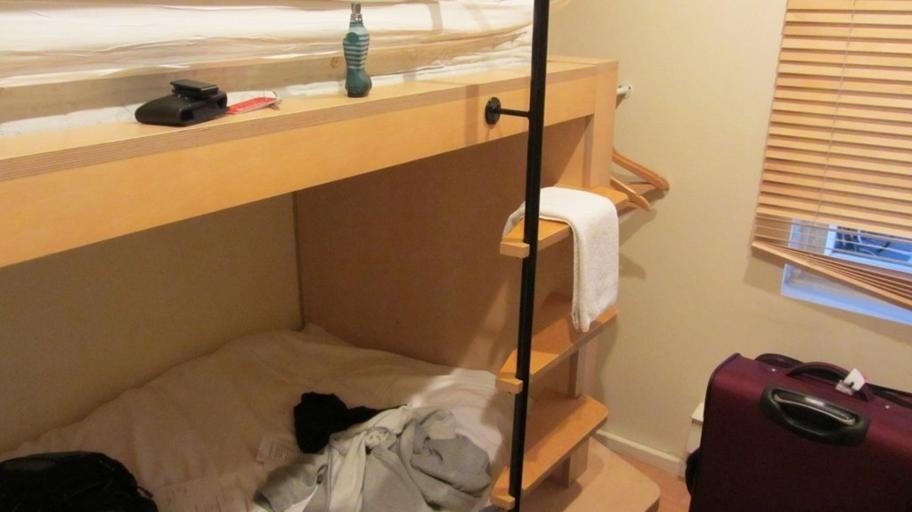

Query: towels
501 186 620 333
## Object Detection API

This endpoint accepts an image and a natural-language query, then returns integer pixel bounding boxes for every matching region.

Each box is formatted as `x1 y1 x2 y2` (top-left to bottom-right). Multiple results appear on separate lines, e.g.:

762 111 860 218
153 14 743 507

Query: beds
0 0 669 511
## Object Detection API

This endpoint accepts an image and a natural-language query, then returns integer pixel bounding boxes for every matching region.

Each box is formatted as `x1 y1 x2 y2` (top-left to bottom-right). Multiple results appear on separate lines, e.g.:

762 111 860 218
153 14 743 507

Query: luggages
683 353 912 512
